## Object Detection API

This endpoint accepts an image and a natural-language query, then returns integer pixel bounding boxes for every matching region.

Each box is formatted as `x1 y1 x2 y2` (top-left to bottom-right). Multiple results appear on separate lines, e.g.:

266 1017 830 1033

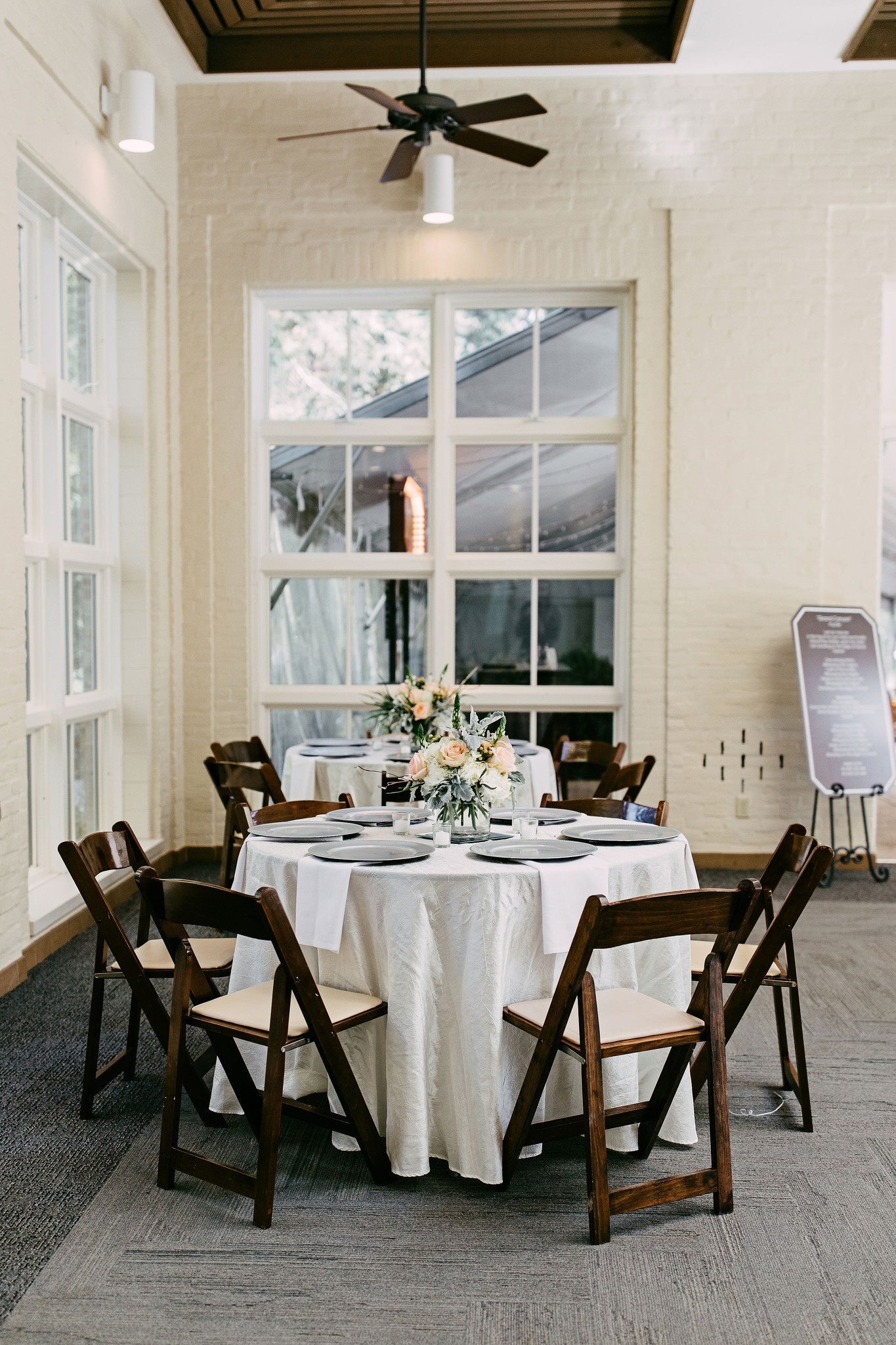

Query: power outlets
736 797 750 819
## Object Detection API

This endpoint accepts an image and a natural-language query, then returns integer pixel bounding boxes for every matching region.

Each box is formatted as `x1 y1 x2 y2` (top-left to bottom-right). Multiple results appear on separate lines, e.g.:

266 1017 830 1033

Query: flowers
359 690 527 828
361 660 478 734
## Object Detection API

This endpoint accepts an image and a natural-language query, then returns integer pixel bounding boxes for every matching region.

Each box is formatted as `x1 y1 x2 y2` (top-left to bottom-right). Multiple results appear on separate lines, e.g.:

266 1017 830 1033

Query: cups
512 810 530 833
520 818 539 839
433 823 451 848
392 812 410 834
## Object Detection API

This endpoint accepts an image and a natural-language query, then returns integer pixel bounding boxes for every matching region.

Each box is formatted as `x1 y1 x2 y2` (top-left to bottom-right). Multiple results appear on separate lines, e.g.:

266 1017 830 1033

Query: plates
308 839 436 860
470 839 599 859
508 739 528 745
247 822 364 838
327 806 434 822
385 752 416 761
299 747 367 756
561 824 680 842
513 748 536 755
306 737 367 747
491 807 582 821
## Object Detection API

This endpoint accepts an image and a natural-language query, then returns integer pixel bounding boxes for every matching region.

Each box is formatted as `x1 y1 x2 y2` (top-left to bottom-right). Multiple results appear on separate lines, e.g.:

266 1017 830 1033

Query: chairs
58 734 836 1249
556 648 613 685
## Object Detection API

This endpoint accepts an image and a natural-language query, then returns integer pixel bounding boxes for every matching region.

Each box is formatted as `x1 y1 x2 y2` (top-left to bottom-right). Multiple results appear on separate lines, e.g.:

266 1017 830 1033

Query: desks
476 660 573 686
204 807 704 1187
281 731 558 808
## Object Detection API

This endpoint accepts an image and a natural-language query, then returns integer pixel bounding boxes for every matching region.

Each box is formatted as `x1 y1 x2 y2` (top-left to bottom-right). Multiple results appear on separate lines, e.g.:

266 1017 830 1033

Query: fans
276 0 549 184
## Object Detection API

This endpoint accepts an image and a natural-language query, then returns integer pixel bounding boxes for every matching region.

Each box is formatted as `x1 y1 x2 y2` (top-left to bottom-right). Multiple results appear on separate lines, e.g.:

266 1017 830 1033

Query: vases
400 726 440 755
437 801 491 842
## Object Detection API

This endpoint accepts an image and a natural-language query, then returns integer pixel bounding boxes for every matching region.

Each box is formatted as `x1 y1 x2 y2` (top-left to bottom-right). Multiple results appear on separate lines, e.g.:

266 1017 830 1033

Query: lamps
377 471 425 686
99 69 155 153
421 153 455 225
